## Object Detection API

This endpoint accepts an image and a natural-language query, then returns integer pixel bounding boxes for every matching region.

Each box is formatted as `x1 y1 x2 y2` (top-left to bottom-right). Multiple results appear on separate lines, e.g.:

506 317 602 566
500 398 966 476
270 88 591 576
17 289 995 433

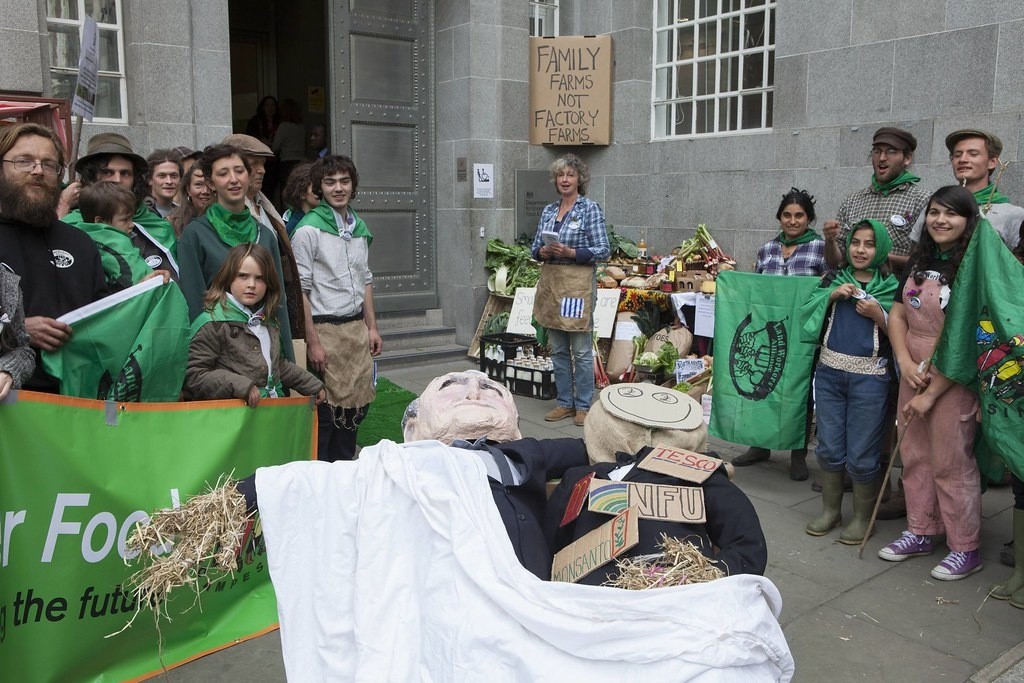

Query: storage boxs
480 332 557 401
467 292 515 359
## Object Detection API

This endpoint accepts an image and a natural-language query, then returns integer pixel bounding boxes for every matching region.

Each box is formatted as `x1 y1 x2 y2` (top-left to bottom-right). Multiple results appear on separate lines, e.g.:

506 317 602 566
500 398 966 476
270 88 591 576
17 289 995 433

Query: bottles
484 345 556 397
637 230 647 257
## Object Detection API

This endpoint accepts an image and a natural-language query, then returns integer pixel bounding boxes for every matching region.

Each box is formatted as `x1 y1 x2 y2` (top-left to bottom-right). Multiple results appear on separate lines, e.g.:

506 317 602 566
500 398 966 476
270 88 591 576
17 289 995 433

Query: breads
599 266 668 287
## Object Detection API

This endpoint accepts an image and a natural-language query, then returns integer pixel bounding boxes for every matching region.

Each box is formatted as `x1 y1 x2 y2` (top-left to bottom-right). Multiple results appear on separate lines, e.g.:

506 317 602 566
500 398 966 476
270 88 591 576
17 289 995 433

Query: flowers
906 288 921 297
903 211 913 221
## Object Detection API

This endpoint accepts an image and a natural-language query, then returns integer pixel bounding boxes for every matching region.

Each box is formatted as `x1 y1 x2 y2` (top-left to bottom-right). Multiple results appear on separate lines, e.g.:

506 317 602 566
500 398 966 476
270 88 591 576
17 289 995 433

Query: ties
474 442 514 487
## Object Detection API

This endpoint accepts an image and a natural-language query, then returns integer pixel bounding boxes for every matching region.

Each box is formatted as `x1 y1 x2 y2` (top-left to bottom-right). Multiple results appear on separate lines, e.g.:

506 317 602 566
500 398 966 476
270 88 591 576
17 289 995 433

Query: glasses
871 148 906 157
2 156 62 175
849 239 876 248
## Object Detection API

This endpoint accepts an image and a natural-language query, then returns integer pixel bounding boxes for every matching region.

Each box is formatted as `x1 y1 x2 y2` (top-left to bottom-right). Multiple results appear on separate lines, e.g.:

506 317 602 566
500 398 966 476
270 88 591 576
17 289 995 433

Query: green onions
473 312 507 357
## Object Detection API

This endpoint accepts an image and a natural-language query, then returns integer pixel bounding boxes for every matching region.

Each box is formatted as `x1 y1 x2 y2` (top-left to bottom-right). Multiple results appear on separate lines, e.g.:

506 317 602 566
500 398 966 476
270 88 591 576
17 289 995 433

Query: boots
805 467 845 536
732 446 769 465
838 470 875 544
791 413 813 481
989 507 1024 610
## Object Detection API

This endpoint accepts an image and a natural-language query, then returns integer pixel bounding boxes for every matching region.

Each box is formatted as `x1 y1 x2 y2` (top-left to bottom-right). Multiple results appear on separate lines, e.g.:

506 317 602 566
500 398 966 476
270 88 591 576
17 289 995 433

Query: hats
172 146 203 162
222 133 275 157
872 127 917 152
946 128 1002 157
74 133 148 177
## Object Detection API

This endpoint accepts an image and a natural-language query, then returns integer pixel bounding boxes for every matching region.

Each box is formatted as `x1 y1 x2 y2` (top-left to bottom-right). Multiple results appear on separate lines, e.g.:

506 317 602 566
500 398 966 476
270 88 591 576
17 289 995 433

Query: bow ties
615 445 647 469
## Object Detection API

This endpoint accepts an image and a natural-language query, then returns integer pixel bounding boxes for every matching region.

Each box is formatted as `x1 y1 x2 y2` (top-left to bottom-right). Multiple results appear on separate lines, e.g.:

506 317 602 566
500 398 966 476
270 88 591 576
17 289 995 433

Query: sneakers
545 405 575 421
931 550 983 581
575 410 588 425
879 529 935 561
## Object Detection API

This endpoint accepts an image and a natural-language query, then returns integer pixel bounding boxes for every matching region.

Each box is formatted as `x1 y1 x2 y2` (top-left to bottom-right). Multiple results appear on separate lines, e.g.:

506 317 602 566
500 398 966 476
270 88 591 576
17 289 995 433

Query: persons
0 96 382 463
131 369 768 683
531 154 610 426
731 127 1024 610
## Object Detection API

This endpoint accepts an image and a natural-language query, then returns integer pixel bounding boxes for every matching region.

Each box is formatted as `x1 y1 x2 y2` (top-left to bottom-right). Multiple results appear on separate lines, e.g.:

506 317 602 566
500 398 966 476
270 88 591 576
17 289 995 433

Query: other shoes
1002 538 1015 566
869 477 907 520
873 472 892 500
813 465 852 492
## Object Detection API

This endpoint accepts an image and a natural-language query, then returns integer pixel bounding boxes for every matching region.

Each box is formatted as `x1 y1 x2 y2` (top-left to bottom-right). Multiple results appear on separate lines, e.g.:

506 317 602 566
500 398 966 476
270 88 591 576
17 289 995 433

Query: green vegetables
592 331 692 394
485 224 638 293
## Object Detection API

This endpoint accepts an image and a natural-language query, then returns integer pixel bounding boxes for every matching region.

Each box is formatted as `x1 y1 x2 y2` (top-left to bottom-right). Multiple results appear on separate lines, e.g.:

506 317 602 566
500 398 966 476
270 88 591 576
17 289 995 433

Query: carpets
355 376 419 448
